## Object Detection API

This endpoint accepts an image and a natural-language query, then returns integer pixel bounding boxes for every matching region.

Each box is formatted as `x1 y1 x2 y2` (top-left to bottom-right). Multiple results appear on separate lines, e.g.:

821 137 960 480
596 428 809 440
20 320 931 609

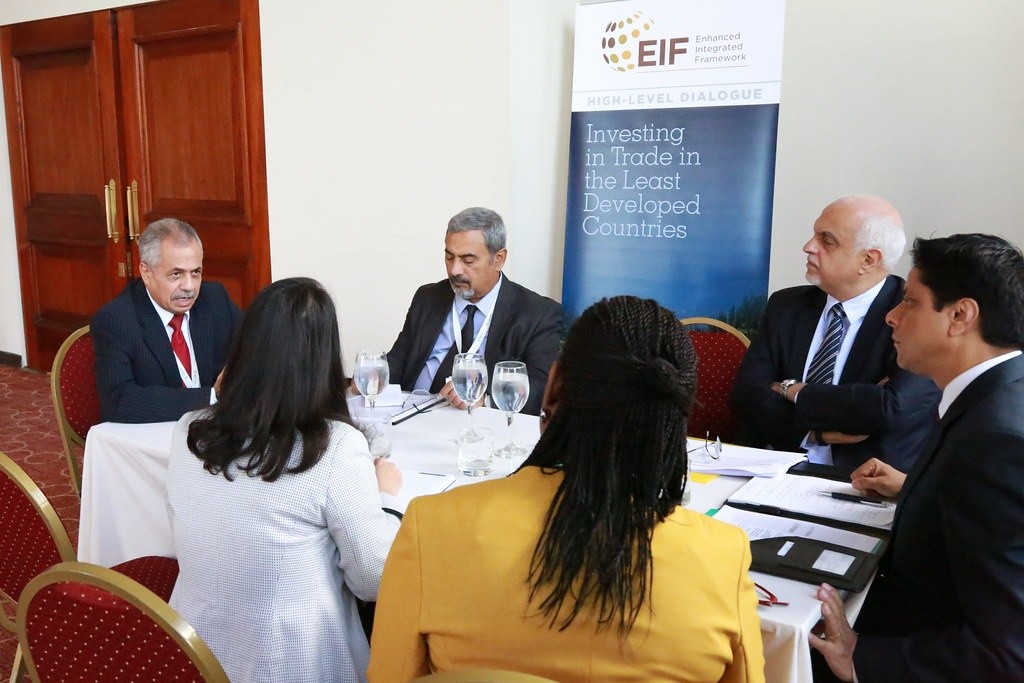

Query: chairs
679 318 751 440
51 325 101 500
0 451 231 683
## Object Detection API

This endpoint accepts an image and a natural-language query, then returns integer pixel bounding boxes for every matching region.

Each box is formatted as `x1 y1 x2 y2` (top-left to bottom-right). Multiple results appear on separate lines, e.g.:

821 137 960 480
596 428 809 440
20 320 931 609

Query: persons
805 233 1024 683
365 295 767 682
86 219 246 425
725 195 945 466
353 209 561 417
166 278 403 683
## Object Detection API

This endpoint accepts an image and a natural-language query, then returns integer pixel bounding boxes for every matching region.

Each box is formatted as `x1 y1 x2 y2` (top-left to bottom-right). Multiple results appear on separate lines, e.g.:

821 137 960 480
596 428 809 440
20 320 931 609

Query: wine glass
353 351 390 424
493 359 528 459
452 352 488 443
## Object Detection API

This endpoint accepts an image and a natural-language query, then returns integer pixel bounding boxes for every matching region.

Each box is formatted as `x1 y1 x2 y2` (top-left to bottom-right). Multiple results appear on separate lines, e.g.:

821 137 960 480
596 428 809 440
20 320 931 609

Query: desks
344 385 897 683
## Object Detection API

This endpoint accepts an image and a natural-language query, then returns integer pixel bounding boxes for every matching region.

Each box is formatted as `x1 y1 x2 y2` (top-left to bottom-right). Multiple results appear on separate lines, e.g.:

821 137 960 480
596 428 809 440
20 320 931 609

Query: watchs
780 378 797 398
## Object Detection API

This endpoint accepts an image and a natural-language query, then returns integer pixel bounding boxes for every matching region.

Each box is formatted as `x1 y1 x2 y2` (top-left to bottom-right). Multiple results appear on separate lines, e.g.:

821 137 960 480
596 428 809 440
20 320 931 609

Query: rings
830 633 841 639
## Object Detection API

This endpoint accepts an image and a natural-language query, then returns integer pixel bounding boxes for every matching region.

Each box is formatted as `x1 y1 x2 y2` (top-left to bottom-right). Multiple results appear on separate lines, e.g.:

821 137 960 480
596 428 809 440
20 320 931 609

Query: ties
168 313 192 390
429 304 478 395
805 302 846 386
932 411 941 426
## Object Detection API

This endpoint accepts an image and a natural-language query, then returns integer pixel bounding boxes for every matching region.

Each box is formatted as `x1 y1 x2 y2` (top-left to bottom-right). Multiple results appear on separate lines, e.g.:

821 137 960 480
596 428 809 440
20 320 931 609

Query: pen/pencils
385 454 391 459
818 490 890 508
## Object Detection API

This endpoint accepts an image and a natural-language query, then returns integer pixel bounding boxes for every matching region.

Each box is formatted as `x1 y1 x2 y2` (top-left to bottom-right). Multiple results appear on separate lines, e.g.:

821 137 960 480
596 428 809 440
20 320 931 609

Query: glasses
753 579 790 608
687 429 722 460
402 389 453 414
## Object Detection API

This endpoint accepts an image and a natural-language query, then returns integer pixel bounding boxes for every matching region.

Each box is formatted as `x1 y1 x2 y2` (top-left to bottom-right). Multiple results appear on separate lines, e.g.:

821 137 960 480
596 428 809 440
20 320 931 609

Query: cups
459 428 495 477
